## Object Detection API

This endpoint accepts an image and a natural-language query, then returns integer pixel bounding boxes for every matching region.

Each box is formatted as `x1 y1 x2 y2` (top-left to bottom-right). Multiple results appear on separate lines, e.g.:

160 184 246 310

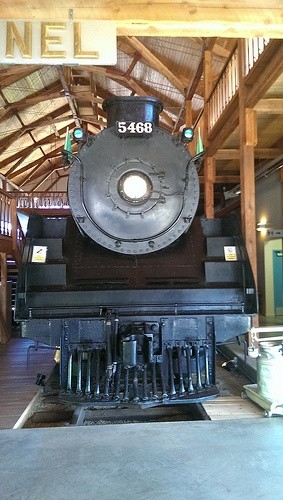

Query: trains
13 97 258 410
